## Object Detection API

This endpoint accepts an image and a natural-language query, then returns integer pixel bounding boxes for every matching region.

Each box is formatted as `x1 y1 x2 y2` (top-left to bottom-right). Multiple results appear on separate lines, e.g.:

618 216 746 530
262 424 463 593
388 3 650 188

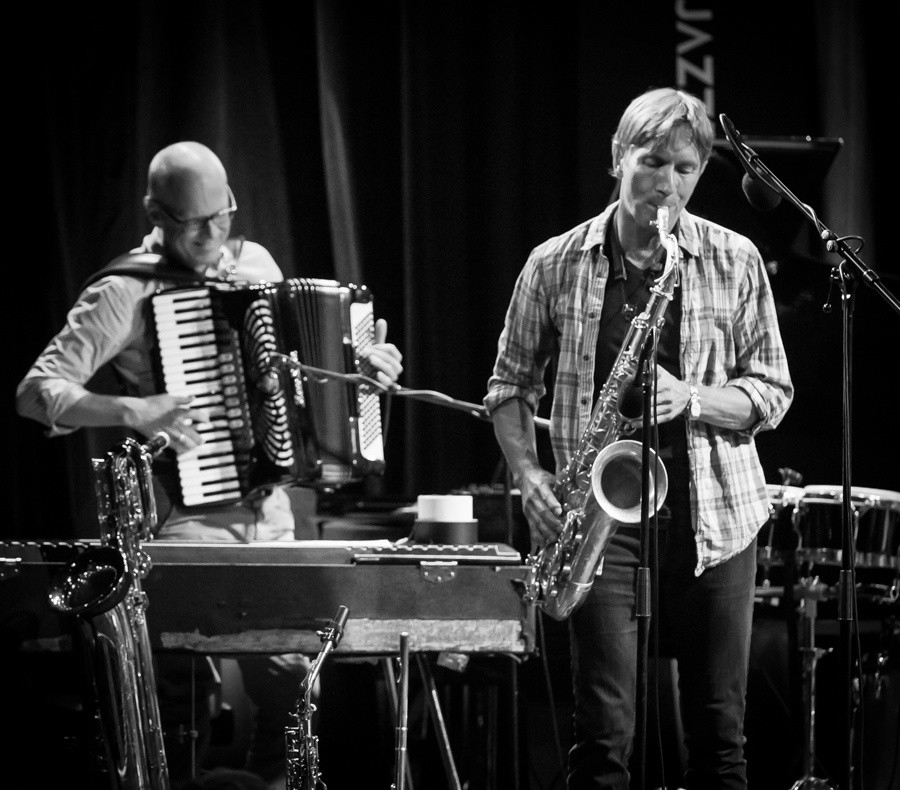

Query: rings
174 433 185 442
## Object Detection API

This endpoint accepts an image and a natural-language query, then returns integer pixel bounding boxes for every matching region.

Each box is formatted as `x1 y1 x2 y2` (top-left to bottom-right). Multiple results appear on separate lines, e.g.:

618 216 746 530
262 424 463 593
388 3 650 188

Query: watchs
682 385 701 421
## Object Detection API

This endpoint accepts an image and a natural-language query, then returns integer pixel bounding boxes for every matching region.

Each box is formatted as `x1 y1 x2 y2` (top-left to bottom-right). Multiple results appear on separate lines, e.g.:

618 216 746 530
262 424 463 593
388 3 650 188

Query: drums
751 483 802 609
796 484 900 608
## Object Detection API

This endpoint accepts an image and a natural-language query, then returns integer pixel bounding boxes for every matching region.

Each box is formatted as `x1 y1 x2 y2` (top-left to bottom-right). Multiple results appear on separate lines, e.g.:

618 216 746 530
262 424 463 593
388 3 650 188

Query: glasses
150 185 237 231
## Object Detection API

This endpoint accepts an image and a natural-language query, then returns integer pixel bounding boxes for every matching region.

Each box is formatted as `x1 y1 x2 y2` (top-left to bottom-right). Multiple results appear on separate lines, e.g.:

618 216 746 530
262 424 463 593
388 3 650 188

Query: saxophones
44 430 174 790
530 203 684 624
282 603 351 790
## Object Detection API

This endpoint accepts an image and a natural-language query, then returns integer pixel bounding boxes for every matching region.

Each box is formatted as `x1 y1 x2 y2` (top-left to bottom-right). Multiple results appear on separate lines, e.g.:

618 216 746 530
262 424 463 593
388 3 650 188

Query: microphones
617 327 659 419
257 356 285 396
719 114 782 210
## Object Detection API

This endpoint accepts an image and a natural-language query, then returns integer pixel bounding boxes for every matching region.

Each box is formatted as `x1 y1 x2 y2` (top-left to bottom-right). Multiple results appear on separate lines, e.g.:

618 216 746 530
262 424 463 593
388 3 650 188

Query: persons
17 143 403 790
484 89 795 790
